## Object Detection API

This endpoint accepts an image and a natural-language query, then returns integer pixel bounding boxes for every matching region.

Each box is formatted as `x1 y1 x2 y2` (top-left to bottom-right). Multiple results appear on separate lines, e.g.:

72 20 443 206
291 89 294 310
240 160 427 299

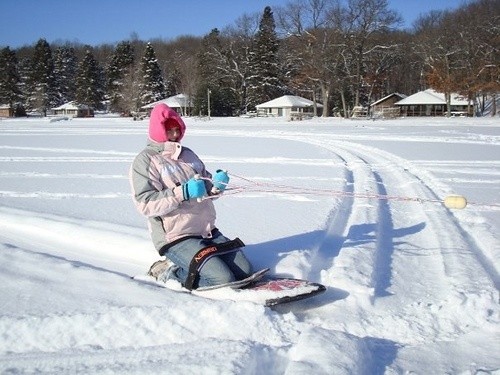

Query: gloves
211 169 229 194
183 174 206 201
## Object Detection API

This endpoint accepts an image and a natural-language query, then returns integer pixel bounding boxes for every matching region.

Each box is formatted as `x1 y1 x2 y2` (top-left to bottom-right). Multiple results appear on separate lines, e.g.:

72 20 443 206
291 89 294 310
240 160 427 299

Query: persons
130 102 253 288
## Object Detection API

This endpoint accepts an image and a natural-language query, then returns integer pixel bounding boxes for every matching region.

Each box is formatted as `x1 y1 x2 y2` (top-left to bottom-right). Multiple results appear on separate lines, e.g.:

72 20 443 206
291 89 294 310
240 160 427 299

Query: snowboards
133 269 325 305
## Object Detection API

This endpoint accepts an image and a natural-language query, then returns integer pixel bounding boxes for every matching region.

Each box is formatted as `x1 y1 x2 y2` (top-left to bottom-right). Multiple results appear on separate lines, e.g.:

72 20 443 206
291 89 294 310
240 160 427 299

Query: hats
165 118 180 129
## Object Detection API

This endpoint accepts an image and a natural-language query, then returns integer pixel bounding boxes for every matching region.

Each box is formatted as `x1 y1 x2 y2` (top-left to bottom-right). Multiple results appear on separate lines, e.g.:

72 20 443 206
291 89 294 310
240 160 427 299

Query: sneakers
148 258 176 280
